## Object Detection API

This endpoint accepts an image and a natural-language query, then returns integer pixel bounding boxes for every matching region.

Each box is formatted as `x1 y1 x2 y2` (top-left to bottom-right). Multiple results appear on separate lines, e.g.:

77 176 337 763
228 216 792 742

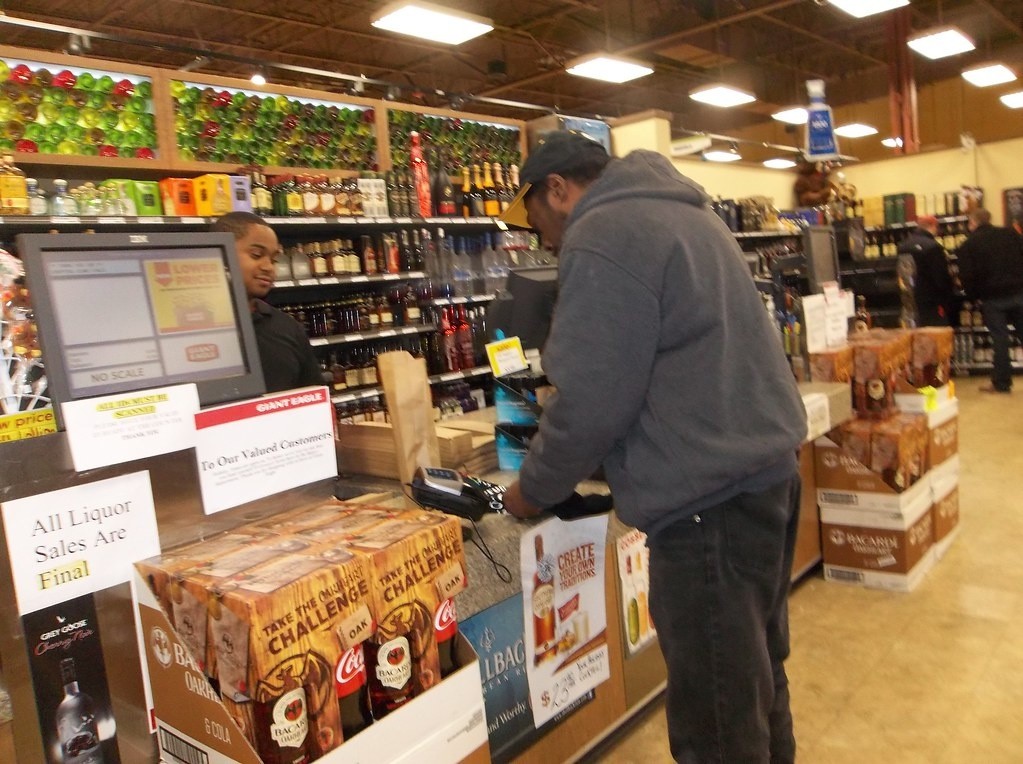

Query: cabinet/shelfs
0 45 528 424
716 214 1023 370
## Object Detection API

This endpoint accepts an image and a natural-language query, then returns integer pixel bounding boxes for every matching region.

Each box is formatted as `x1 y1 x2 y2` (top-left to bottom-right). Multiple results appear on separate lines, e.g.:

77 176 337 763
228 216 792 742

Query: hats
497 130 608 228
917 216 938 225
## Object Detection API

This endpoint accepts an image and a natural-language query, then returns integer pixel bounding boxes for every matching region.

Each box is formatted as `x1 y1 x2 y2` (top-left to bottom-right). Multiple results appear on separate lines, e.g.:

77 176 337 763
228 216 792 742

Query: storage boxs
814 386 960 591
132 560 491 763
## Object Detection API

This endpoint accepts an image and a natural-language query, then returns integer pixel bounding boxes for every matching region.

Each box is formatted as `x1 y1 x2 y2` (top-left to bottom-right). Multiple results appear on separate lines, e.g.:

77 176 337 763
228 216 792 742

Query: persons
496 132 808 764
956 208 1023 394
1012 209 1023 237
208 210 324 394
896 214 961 327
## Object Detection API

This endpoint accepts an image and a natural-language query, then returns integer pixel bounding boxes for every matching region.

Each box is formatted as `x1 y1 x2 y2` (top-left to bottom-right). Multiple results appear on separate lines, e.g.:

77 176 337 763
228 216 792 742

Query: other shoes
978 386 1011 394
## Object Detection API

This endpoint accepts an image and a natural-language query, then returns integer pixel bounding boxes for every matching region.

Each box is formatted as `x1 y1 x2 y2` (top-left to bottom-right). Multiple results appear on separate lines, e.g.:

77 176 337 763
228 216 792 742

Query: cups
572 611 592 646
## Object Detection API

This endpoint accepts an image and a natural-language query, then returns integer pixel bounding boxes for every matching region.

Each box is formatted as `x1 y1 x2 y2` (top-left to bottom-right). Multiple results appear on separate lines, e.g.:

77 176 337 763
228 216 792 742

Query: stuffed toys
793 153 831 206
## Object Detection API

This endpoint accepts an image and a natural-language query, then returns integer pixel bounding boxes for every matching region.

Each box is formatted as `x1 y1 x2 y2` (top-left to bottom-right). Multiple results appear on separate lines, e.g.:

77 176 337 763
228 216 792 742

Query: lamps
771 25 807 125
688 0 757 108
704 145 741 162
763 153 797 169
370 0 497 45
564 0 657 84
826 0 1023 138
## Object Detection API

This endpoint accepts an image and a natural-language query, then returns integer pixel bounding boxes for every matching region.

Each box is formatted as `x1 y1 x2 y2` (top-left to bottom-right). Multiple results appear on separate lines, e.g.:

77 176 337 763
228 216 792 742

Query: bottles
260 597 459 764
627 552 655 643
853 294 871 332
57 657 104 764
532 535 558 667
861 222 1022 363
0 153 132 217
249 152 519 424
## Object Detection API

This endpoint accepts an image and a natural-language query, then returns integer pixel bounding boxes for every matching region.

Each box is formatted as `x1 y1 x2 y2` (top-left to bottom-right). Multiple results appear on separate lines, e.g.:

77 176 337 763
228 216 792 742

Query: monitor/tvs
489 267 561 365
16 231 267 434
803 225 842 294
